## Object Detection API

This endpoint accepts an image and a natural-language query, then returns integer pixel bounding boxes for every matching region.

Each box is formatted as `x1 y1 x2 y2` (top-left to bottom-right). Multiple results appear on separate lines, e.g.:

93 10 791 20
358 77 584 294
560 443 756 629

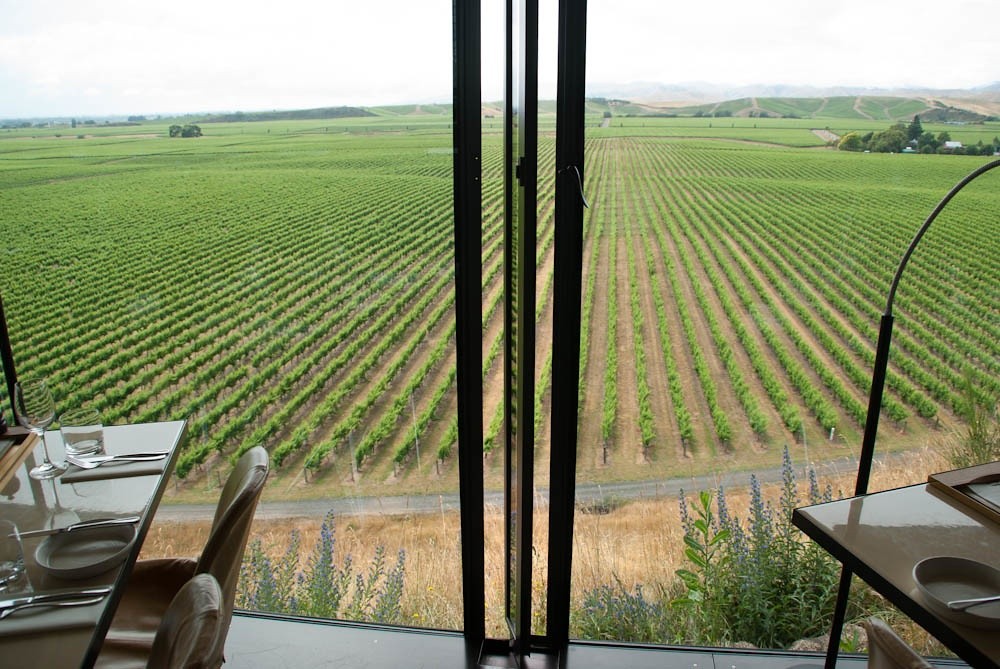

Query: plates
33 518 137 580
912 555 1000 630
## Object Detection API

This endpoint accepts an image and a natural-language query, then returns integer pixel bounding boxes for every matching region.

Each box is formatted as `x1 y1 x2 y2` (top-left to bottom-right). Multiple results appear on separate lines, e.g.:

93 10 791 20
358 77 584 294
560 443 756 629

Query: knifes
7 516 141 538
0 585 112 609
64 449 169 462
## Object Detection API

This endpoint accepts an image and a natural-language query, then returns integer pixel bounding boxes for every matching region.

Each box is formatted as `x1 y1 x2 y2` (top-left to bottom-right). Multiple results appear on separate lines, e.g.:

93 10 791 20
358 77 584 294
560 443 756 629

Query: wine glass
31 472 81 530
14 378 69 477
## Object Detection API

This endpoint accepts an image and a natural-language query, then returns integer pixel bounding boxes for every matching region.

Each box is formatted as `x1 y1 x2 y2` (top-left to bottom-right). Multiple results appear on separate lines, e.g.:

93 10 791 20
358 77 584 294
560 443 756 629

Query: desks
0 419 189 669
792 483 1000 669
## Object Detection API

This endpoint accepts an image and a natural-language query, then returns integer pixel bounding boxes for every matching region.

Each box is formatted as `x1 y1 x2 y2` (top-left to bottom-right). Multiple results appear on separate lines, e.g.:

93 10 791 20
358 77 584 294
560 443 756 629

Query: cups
0 575 34 606
0 519 34 587
58 407 107 459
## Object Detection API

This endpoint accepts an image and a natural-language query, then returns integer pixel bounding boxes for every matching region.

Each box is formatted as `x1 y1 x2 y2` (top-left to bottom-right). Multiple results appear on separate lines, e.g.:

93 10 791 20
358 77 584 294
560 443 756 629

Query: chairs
106 446 269 669
866 616 932 669
93 574 222 669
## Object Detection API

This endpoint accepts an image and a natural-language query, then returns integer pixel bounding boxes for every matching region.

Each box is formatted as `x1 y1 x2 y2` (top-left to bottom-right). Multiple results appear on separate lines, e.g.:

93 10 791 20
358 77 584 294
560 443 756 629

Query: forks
66 455 166 469
